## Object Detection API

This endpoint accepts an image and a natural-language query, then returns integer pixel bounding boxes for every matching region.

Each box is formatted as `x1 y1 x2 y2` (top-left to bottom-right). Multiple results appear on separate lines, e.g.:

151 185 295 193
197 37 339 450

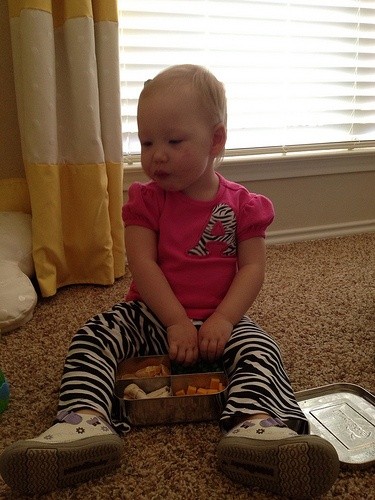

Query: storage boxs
116 353 227 428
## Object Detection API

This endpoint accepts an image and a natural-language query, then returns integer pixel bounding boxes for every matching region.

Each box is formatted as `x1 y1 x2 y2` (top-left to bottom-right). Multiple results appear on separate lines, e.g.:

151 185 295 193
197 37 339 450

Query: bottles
0 369 9 413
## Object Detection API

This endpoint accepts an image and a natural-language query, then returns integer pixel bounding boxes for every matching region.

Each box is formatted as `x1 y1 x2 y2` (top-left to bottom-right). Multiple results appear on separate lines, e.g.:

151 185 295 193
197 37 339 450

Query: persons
1 64 340 500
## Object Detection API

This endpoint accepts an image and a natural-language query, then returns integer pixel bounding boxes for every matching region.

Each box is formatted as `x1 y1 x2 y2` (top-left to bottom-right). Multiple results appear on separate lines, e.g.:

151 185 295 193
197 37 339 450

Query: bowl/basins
112 354 229 426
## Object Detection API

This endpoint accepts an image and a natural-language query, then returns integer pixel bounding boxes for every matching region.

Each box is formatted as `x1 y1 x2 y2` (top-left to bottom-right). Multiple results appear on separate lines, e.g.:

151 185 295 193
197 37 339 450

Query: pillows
0 209 33 333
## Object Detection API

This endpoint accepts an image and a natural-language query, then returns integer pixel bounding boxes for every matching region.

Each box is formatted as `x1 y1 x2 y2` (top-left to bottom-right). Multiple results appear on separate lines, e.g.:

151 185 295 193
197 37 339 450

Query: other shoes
216 417 340 499
0 410 126 496
